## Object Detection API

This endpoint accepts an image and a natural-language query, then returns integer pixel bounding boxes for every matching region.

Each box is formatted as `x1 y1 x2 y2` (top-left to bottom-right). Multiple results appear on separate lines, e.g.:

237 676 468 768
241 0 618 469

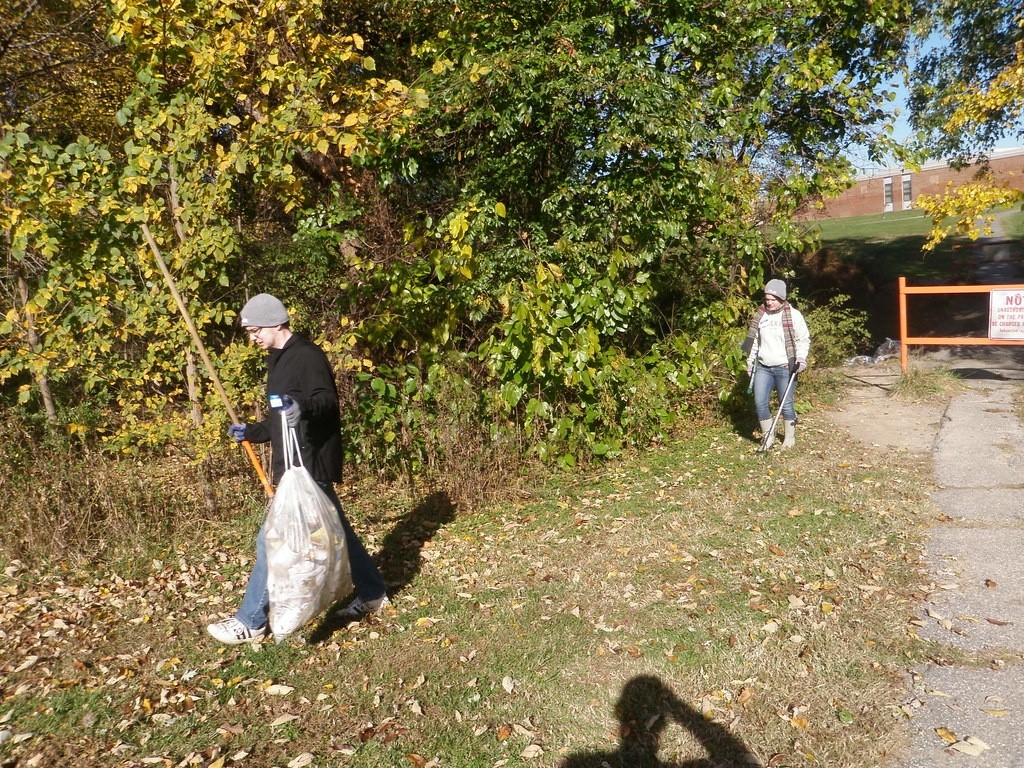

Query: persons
207 293 392 645
746 279 811 451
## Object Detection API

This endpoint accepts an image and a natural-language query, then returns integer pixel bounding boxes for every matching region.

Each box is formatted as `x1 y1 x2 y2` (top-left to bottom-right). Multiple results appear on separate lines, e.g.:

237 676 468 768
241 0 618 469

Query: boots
756 417 776 451
779 420 795 450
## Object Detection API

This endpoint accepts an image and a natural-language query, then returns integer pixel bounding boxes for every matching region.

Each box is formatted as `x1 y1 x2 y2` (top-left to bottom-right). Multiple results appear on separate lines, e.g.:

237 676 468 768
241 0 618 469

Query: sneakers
207 618 266 644
336 590 393 619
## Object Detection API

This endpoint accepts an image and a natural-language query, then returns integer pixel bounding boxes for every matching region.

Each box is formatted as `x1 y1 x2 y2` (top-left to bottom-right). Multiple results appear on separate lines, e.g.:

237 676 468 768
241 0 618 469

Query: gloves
278 399 302 428
746 364 754 377
228 421 247 442
795 357 807 373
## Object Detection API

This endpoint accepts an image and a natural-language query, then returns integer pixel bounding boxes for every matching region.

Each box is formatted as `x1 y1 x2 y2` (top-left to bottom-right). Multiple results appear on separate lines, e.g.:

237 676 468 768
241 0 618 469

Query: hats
240 292 290 327
764 279 787 300
